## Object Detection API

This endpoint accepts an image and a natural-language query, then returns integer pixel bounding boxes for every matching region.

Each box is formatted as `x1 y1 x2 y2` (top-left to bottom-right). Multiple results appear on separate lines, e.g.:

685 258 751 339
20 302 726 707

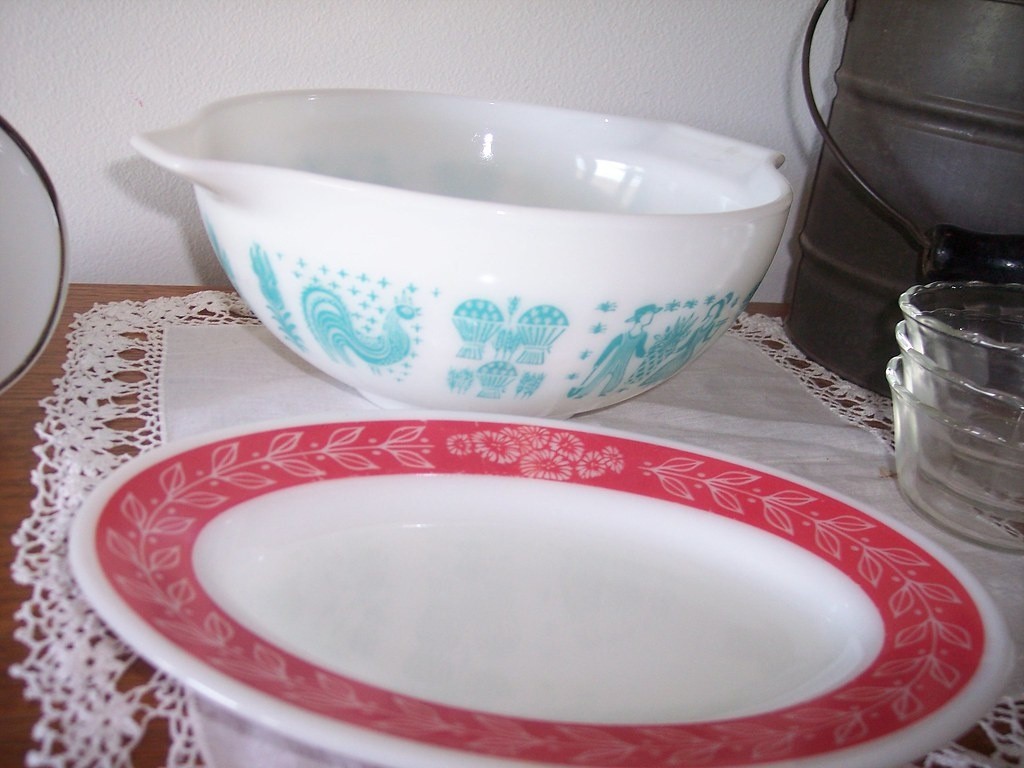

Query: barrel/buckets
782 1 1024 397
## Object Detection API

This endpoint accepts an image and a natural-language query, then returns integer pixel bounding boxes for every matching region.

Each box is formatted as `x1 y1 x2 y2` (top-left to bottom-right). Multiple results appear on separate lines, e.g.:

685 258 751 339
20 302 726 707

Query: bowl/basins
887 281 1024 555
137 90 791 420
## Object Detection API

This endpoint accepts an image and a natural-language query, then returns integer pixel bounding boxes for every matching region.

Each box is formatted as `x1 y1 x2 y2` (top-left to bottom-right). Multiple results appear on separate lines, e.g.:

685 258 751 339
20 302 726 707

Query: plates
66 411 1013 768
0 115 70 395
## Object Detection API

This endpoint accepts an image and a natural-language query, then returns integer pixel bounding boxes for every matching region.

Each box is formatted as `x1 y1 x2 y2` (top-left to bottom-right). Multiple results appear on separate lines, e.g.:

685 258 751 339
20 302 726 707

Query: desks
0 284 1024 768
